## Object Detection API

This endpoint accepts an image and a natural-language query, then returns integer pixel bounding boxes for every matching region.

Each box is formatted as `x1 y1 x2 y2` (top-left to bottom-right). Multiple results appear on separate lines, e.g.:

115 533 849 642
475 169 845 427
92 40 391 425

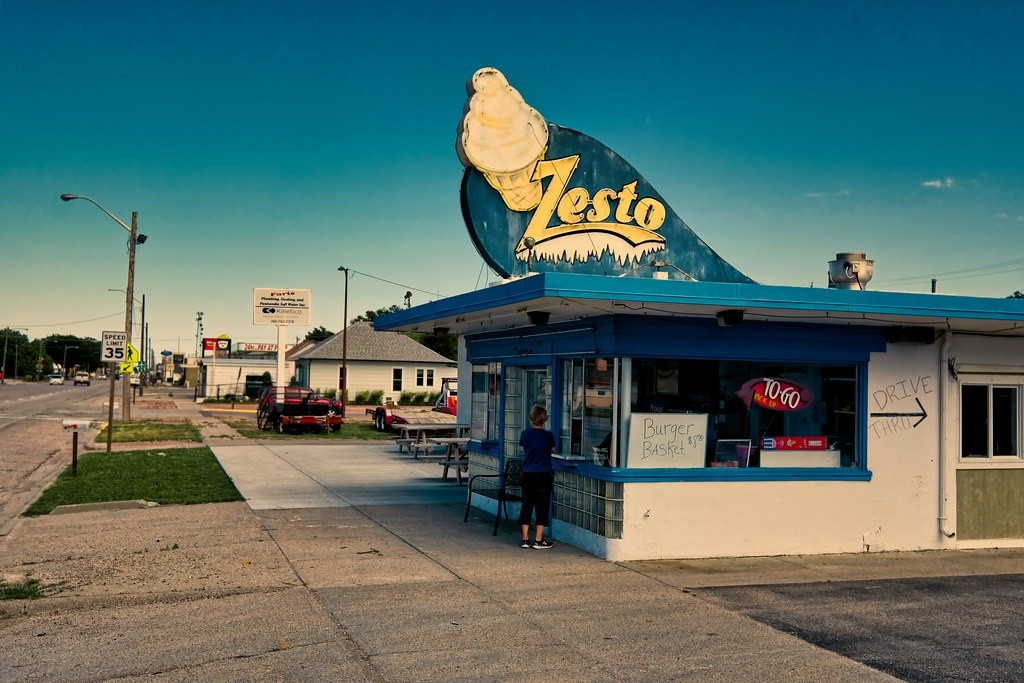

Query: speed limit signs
99 331 127 363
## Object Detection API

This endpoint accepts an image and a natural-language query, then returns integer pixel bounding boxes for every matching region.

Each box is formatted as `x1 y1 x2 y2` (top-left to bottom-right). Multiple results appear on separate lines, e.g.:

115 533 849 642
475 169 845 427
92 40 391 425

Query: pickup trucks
73 371 90 386
259 385 343 434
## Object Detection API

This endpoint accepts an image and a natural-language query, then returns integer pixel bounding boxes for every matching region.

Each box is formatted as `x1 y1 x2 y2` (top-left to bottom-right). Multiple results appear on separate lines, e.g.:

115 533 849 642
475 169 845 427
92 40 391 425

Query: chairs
462 457 523 537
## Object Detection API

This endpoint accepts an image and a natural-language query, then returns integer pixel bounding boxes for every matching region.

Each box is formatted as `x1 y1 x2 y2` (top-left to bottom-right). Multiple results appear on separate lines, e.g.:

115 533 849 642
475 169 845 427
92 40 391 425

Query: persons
518 405 557 551
596 404 620 467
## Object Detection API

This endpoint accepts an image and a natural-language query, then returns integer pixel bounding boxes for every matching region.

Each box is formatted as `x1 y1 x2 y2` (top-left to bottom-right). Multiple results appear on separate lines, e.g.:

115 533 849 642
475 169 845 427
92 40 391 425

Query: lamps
526 311 553 326
717 309 744 328
431 326 451 338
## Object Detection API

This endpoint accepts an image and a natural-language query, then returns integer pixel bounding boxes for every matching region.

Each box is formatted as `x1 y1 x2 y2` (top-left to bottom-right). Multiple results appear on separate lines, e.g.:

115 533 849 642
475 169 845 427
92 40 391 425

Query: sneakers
521 538 531 548
533 538 554 549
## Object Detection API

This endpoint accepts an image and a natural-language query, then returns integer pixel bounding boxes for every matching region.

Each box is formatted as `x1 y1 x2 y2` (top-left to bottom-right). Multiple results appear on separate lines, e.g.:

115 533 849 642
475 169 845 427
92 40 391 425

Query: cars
98 373 107 380
49 374 65 386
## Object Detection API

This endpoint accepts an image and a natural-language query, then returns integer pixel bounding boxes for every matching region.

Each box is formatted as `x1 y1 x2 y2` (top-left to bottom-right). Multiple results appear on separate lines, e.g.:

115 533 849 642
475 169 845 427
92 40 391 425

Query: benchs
411 441 467 449
437 461 469 467
394 438 469 444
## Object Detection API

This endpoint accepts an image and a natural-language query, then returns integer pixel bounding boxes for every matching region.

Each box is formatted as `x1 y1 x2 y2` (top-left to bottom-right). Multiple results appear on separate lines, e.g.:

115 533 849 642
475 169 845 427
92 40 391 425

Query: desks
392 423 463 459
427 436 470 487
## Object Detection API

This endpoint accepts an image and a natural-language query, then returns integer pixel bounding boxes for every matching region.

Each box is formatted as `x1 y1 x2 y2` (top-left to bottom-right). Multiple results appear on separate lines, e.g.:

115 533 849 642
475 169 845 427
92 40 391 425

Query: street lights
62 344 79 375
59 192 138 423
36 339 57 382
337 265 350 390
107 288 146 364
2 327 29 384
